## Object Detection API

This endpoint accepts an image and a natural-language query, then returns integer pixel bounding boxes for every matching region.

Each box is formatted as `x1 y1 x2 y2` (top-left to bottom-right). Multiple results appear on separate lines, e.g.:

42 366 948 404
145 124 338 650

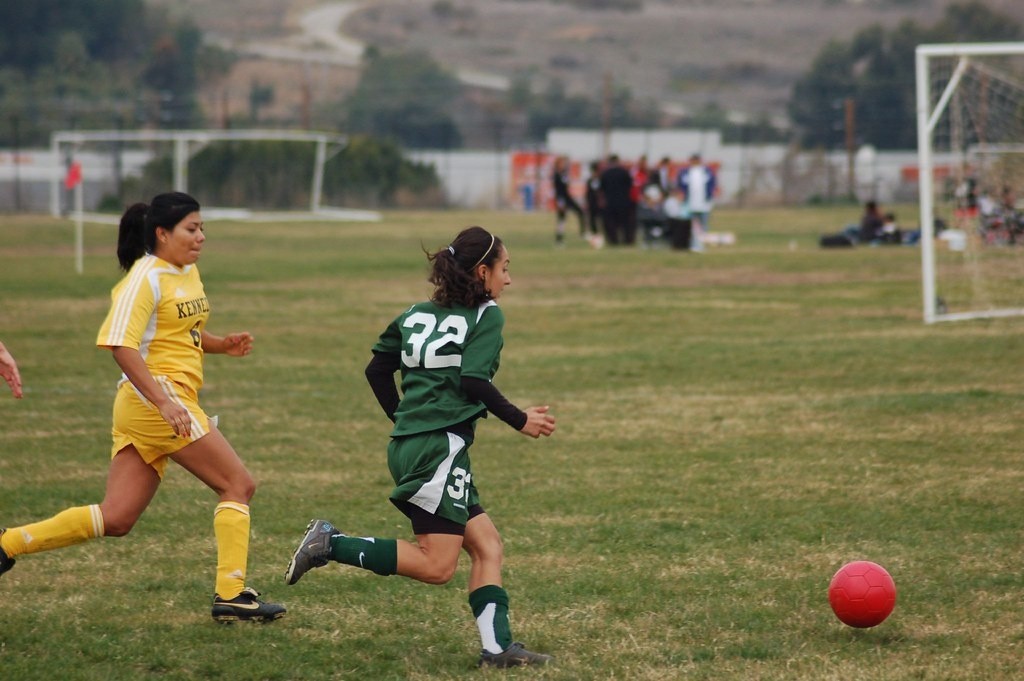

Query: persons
0 341 24 399
0 192 287 625
284 225 557 668
551 152 718 252
819 157 1024 249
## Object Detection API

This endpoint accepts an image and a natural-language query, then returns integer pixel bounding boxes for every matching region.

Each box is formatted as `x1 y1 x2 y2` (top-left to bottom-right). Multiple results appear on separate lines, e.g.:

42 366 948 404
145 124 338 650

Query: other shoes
479 641 555 668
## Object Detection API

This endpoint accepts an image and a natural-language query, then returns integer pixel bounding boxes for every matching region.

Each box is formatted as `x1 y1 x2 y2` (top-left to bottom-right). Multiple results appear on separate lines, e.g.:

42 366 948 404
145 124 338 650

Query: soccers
827 559 899 630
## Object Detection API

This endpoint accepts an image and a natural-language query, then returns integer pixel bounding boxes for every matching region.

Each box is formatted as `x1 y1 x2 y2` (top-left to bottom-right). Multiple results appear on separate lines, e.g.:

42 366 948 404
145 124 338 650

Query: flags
64 159 82 189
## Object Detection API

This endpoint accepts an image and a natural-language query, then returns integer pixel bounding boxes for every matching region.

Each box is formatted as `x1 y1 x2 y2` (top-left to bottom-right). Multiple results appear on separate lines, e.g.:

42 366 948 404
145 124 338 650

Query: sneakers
283 518 339 585
212 587 286 625
0 529 14 577
